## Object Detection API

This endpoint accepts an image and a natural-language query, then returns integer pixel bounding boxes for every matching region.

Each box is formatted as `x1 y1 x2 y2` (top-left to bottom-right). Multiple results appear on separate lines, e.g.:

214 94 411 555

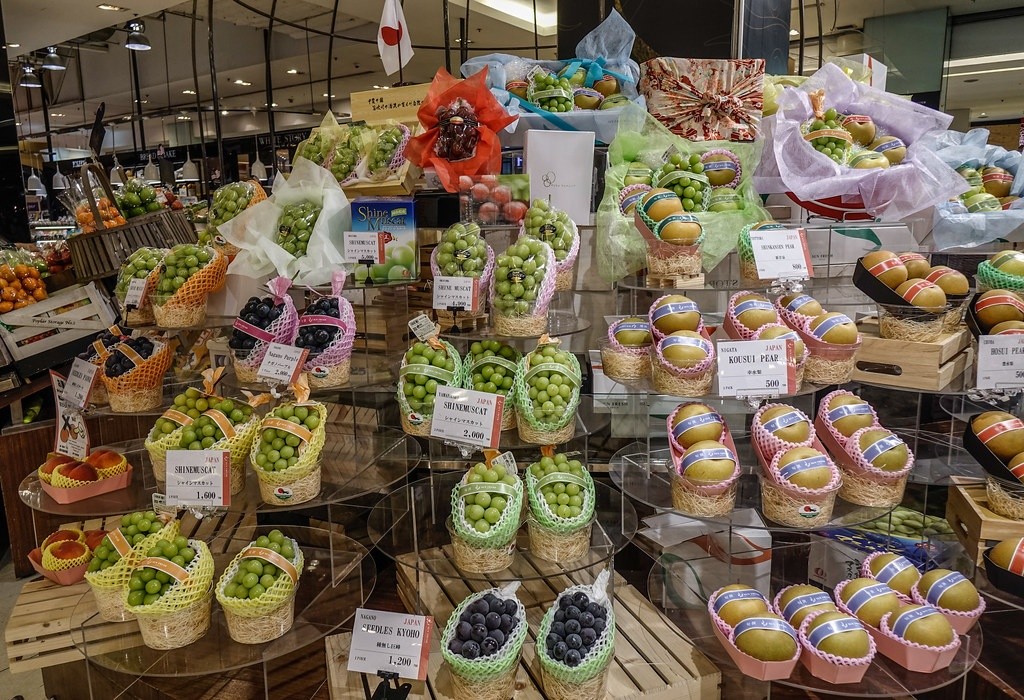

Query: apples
614 292 906 489
844 116 905 167
506 66 628 109
712 554 979 659
949 165 1020 213
620 153 788 246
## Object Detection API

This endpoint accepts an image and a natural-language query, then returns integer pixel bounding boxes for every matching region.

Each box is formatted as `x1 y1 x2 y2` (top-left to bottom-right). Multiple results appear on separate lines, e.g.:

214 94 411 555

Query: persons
157 143 166 155
210 169 222 180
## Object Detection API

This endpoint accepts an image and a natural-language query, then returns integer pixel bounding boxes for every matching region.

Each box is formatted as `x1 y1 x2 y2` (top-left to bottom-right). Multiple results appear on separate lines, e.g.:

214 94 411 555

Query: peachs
41 449 127 571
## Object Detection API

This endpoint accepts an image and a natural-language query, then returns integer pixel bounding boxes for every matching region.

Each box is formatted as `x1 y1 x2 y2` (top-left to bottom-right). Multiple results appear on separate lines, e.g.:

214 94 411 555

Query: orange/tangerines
0 179 160 314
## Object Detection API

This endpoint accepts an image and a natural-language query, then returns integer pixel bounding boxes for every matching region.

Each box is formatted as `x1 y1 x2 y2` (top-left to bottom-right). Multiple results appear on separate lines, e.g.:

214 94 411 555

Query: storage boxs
853 316 973 391
933 143 1024 254
640 59 764 142
351 195 421 281
788 221 921 278
520 128 596 226
807 502 975 590
654 510 773 608
0 278 118 361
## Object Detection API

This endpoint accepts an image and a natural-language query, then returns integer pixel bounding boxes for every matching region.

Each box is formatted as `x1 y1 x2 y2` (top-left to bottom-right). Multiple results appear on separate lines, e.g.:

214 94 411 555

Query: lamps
250 106 268 181
41 45 67 71
28 146 42 190
180 111 200 181
110 121 125 185
144 147 161 184
20 57 41 87
124 17 151 51
36 152 48 197
52 119 70 189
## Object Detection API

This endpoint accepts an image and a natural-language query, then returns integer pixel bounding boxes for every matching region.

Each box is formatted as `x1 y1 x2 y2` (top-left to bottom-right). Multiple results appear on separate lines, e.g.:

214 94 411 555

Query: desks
18 147 1024 700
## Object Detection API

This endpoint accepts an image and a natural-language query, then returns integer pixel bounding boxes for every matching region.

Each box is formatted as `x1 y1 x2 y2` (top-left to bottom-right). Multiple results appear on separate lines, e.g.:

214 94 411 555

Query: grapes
658 154 707 212
808 108 848 163
85 387 319 606
403 199 589 528
458 175 528 222
294 106 477 182
76 183 341 378
533 73 572 112
445 590 609 668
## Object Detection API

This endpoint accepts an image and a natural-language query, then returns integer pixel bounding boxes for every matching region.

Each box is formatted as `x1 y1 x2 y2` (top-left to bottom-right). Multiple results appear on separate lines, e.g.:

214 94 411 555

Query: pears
861 250 1024 337
972 412 1024 484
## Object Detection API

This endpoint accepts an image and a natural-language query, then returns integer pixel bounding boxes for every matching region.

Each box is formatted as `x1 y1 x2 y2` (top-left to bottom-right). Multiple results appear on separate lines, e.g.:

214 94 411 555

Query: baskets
65 164 198 282
